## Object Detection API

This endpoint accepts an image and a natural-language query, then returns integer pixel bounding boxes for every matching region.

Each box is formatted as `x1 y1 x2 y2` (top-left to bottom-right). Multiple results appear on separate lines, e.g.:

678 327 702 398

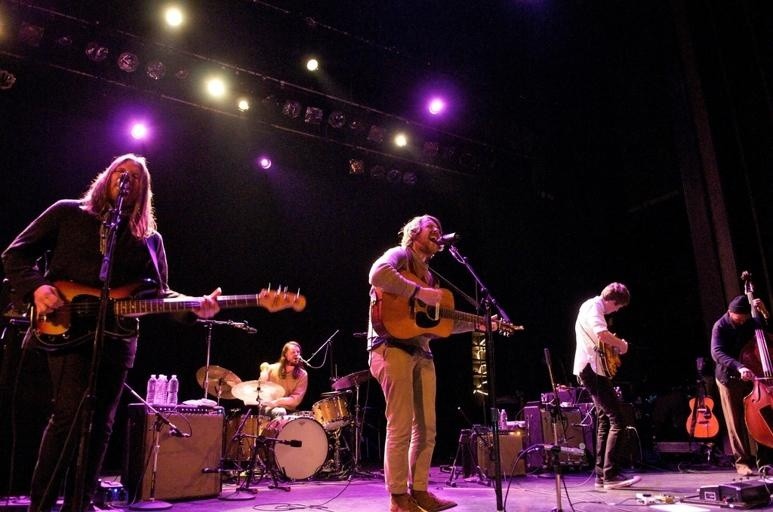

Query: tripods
328 391 375 482
444 427 493 488
221 413 270 486
676 356 736 472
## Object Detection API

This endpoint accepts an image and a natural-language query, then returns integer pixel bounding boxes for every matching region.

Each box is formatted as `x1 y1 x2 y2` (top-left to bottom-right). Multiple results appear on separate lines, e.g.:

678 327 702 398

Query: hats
728 295 750 314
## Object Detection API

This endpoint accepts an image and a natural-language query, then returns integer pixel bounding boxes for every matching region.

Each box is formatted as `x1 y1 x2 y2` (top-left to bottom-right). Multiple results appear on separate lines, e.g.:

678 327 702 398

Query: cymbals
231 380 285 403
333 370 371 390
196 365 242 399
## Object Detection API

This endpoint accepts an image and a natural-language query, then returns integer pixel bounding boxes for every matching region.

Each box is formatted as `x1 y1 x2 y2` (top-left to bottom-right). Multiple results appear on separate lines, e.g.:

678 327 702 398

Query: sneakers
595 477 603 486
410 489 457 512
389 494 428 512
603 472 642 489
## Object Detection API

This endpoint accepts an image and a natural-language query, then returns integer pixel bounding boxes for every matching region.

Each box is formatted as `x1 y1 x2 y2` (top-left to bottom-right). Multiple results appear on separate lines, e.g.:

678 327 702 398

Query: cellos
742 271 773 448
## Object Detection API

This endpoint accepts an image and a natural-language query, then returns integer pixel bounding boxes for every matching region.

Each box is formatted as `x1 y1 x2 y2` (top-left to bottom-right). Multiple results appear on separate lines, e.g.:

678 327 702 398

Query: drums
258 416 330 481
312 397 351 430
224 416 271 461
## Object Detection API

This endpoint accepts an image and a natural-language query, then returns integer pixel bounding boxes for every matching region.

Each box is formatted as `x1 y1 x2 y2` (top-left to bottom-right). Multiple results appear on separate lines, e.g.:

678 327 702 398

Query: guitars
687 358 719 438
32 275 306 351
371 270 524 346
598 338 621 379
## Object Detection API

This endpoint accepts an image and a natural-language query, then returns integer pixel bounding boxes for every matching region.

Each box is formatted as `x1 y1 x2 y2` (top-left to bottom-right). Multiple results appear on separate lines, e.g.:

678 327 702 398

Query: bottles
167 375 178 405
501 409 508 431
146 374 157 403
164 375 168 394
155 374 167 404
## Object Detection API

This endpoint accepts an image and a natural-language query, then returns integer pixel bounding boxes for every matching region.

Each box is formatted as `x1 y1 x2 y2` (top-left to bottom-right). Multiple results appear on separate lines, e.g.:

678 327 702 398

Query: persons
0 154 221 512
369 215 498 512
260 341 308 420
573 283 642 490
710 295 771 475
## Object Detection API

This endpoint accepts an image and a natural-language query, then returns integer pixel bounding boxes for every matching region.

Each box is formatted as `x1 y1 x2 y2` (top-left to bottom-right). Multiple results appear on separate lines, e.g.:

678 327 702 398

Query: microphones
435 232 460 248
117 170 131 186
